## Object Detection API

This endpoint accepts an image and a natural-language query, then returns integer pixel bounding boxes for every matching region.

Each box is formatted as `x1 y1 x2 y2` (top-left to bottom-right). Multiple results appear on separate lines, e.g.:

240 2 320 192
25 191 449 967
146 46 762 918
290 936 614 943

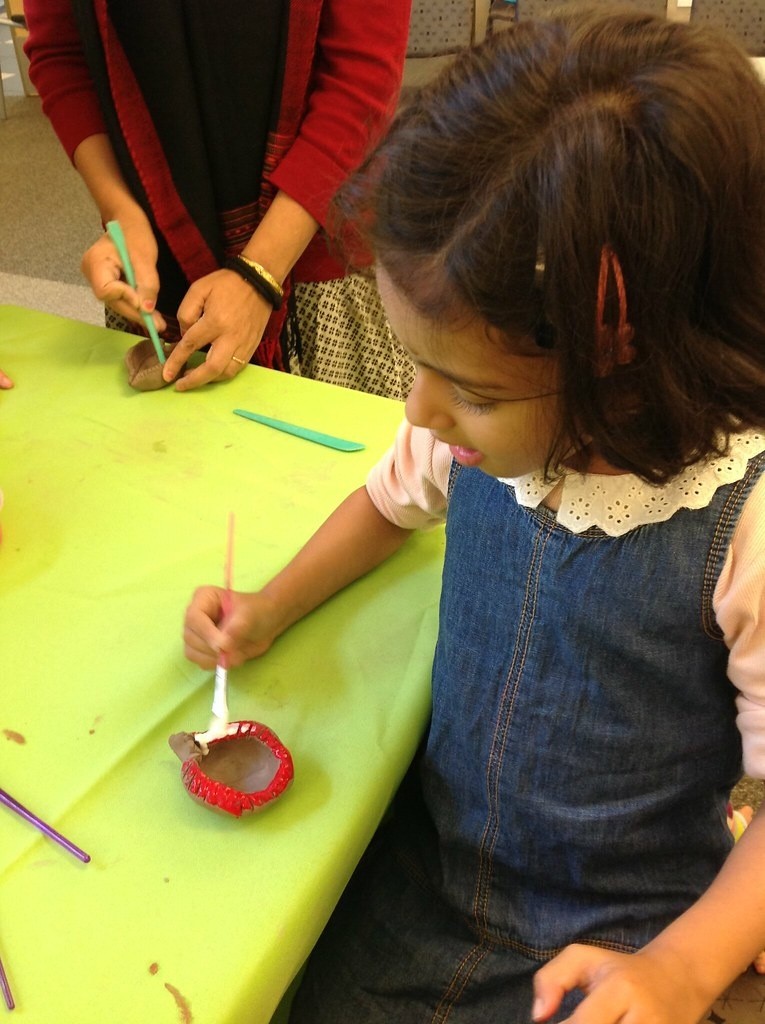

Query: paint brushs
0 788 91 864
208 511 235 738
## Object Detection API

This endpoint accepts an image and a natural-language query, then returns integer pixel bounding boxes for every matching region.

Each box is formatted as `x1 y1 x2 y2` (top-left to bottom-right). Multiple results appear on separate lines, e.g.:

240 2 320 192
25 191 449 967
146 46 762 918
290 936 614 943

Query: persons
24 0 413 393
177 11 765 1024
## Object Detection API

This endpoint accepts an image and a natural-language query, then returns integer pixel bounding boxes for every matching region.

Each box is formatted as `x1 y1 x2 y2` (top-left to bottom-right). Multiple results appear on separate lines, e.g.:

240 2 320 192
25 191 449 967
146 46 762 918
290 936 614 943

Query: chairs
403 0 765 89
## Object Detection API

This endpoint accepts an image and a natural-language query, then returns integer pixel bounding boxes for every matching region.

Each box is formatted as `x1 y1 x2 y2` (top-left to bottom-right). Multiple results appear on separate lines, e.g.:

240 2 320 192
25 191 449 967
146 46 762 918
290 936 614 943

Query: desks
0 303 443 1024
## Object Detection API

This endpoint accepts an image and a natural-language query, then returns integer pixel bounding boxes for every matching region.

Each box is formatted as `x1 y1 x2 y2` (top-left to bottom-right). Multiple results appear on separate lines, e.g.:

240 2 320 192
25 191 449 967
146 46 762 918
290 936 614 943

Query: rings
232 356 246 365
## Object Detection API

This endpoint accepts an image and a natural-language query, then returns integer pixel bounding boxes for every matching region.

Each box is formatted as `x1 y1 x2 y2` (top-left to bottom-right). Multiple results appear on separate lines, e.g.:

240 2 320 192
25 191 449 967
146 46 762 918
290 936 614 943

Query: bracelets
225 254 286 309
